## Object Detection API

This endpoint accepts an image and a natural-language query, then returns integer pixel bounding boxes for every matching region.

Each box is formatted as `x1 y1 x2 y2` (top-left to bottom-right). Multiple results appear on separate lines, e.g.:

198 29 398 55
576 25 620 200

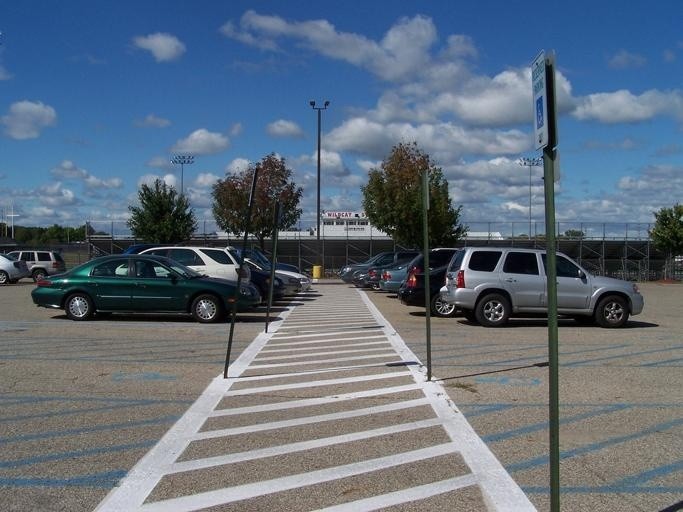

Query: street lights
308 98 333 268
169 154 196 198
517 156 544 239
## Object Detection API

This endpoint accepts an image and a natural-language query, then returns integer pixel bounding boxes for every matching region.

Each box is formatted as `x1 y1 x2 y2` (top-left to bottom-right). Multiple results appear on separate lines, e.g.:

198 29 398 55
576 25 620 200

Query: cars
378 255 416 292
349 267 369 288
231 243 312 303
0 252 28 286
123 244 182 255
368 256 416 286
29 252 264 322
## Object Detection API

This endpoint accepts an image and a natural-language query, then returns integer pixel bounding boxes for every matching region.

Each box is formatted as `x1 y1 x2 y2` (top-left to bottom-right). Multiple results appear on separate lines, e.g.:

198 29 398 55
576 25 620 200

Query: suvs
117 244 254 285
393 248 462 317
435 245 645 329
6 250 66 284
336 250 394 285
